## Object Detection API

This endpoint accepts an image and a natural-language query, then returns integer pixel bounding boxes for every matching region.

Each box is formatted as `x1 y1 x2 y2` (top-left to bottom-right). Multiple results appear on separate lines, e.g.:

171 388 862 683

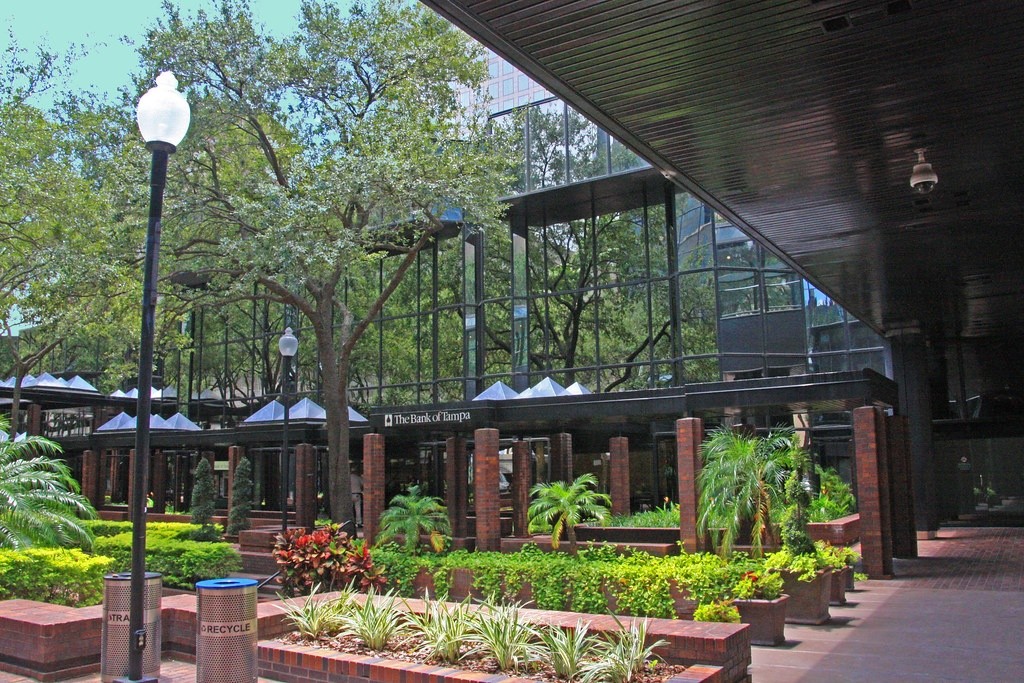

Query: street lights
277 322 299 540
130 70 193 676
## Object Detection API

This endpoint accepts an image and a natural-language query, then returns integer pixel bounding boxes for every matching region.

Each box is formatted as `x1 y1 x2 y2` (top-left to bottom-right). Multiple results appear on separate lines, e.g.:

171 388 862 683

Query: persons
350 468 364 527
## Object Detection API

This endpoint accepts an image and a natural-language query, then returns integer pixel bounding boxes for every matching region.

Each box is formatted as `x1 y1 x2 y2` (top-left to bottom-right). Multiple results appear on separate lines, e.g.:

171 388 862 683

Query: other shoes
358 525 363 528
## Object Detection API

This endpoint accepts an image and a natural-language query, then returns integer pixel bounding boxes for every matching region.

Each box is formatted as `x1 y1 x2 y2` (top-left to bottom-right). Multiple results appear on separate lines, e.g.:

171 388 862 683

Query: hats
351 467 358 471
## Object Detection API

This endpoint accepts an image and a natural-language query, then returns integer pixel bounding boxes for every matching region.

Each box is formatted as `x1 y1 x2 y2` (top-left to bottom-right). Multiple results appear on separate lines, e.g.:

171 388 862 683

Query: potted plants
0 419 858 683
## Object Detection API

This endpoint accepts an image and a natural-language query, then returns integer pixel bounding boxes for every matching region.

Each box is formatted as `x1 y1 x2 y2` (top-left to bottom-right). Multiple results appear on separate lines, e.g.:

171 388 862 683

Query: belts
352 493 361 494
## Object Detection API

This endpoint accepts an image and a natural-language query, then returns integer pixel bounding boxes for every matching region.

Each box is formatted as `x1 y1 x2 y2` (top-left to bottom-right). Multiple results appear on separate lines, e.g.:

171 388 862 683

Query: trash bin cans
194 577 259 683
101 571 164 683
